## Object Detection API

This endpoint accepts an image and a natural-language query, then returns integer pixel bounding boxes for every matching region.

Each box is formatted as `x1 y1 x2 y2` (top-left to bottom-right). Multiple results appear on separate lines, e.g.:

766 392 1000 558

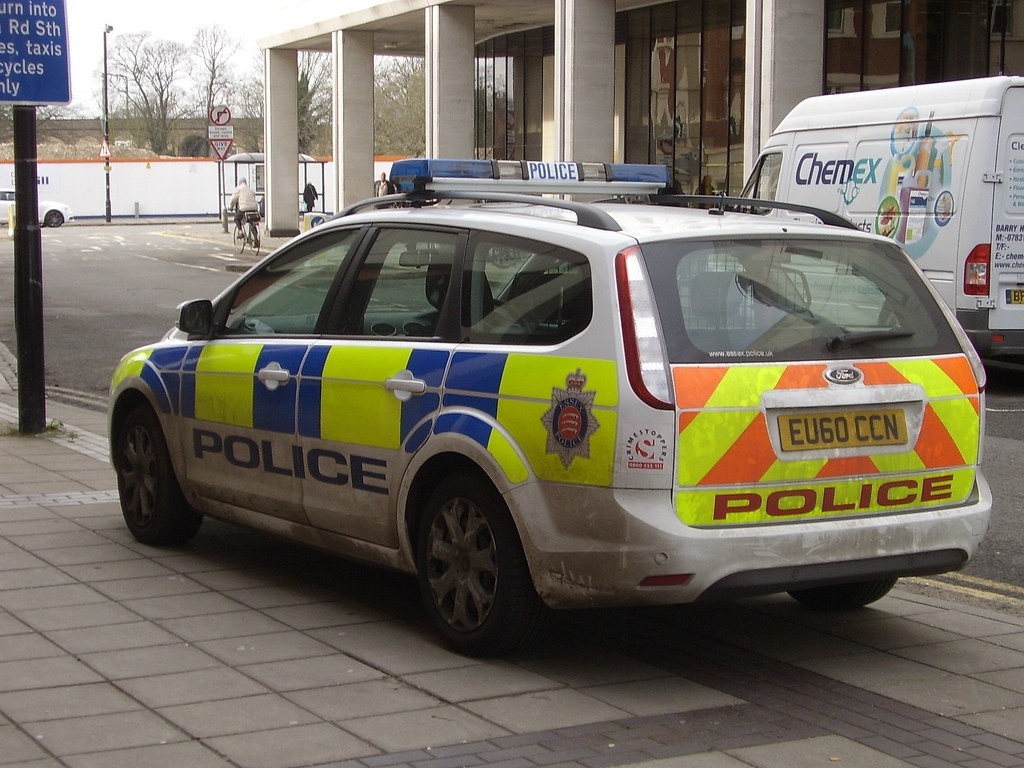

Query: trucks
707 76 1024 356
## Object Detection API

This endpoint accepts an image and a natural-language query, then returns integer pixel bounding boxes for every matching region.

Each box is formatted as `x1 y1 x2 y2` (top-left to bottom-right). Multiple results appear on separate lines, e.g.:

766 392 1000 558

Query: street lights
103 22 113 223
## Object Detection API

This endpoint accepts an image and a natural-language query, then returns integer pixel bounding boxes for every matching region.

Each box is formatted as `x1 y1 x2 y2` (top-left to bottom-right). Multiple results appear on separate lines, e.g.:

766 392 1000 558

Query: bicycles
228 208 260 255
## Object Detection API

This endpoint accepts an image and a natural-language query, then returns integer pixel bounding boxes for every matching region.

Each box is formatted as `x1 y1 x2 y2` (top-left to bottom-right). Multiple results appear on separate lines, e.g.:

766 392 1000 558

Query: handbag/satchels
301 201 308 212
314 200 319 208
245 211 261 222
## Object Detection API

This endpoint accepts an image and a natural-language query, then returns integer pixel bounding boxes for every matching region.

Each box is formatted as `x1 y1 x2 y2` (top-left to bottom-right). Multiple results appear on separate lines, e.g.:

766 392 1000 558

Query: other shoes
254 239 259 248
237 234 245 238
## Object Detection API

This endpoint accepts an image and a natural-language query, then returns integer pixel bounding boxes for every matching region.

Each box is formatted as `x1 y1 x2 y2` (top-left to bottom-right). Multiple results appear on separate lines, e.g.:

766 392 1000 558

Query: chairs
686 272 762 355
424 253 495 317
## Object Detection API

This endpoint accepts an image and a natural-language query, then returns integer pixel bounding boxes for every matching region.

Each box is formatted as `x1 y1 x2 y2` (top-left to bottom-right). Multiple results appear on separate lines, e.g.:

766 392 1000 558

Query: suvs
107 159 993 659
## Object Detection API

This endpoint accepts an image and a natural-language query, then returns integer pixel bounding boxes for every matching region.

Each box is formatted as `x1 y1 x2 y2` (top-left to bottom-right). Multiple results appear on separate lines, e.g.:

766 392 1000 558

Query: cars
0 188 75 227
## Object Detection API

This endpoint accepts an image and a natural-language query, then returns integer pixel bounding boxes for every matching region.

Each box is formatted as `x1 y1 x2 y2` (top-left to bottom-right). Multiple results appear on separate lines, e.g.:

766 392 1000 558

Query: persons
375 173 394 197
303 182 318 211
230 178 260 248
